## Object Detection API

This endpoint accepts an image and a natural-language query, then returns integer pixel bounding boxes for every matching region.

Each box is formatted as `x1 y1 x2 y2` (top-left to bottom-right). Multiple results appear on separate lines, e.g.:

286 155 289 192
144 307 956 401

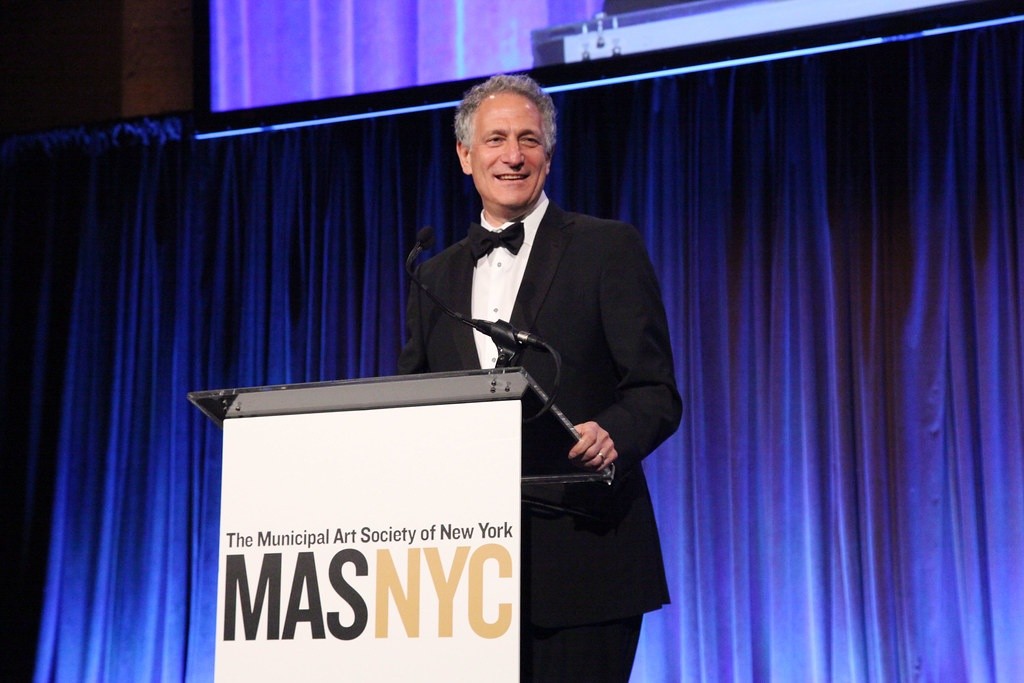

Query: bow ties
468 221 525 260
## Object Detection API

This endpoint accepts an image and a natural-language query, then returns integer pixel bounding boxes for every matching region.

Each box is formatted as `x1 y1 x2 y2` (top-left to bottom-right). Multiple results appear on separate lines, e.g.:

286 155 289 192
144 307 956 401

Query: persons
399 74 684 683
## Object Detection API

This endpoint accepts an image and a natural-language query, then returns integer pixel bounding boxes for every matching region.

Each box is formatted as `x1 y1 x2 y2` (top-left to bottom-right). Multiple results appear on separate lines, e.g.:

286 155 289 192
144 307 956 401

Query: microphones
406 226 549 352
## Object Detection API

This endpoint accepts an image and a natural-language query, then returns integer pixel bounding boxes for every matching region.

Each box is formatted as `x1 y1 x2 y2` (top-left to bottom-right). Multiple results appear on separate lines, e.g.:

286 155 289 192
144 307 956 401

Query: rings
599 452 605 461
